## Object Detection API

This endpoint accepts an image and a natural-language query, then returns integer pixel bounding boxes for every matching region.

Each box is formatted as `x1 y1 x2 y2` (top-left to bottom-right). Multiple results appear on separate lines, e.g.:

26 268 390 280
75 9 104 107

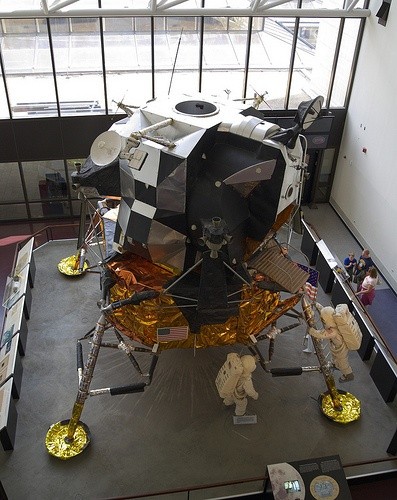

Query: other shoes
338 373 354 383
223 399 233 405
235 410 245 416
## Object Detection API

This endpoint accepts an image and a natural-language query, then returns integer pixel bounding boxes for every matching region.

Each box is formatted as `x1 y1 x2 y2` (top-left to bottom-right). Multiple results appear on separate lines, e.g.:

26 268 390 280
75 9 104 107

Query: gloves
252 392 258 399
309 328 315 334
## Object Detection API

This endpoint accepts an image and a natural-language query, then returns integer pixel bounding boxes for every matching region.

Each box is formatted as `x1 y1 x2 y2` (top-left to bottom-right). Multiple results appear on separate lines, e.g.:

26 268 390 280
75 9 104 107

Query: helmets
241 355 256 371
322 307 334 320
